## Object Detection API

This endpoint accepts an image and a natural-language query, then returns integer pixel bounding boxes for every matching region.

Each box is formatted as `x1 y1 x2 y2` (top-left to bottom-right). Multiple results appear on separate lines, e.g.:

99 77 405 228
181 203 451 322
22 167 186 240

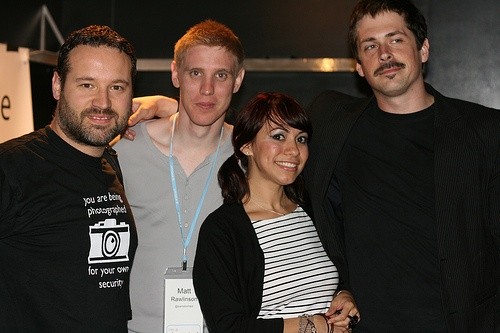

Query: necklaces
251 198 284 216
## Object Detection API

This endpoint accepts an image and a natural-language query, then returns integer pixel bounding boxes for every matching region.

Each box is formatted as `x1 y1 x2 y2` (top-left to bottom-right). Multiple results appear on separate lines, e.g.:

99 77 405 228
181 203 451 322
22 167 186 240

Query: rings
347 315 359 328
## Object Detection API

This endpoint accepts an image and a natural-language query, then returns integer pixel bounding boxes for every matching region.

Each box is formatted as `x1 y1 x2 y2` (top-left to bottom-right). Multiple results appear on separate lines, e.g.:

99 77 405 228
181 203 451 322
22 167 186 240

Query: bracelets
336 291 343 295
298 314 335 333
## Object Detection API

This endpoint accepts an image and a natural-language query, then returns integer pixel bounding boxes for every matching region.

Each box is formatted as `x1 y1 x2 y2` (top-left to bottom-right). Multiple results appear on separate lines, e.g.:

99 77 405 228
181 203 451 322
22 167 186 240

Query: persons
0 26 138 333
110 20 245 333
193 94 361 333
337 2 500 333
121 1 500 333
